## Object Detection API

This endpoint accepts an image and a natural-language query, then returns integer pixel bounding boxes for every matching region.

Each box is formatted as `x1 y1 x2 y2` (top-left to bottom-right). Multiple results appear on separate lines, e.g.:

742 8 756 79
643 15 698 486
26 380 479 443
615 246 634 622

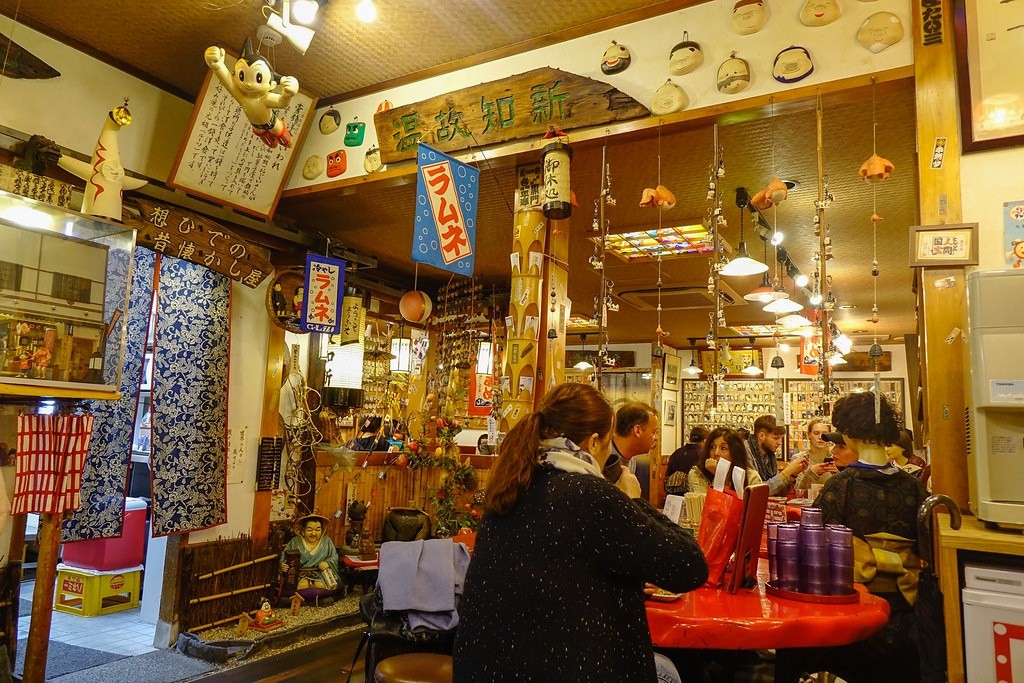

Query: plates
766 580 861 603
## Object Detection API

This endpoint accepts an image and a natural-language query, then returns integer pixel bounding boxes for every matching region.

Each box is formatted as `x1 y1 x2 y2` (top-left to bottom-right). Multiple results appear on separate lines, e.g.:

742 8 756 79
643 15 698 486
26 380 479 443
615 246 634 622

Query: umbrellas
907 493 964 683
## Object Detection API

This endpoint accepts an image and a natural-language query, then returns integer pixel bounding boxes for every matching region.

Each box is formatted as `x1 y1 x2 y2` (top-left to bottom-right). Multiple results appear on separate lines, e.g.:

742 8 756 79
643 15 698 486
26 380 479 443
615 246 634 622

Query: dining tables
644 554 891 651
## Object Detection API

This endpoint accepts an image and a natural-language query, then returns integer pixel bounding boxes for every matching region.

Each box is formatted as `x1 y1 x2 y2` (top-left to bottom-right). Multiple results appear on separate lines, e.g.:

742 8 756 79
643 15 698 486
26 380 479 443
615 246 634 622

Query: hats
821 428 847 445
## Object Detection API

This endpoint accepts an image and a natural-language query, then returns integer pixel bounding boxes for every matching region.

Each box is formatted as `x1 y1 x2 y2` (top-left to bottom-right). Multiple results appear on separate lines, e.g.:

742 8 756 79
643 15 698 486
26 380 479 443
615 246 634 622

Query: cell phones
804 454 809 459
823 458 833 463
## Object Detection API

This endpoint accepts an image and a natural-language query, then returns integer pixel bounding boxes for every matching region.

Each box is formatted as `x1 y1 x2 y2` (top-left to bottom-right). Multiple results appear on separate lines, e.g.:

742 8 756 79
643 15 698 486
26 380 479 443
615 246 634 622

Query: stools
365 630 456 683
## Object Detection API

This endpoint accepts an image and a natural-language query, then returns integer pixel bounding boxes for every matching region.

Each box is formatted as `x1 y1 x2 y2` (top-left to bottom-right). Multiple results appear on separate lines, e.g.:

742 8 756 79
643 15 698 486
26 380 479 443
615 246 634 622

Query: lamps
572 334 593 371
681 185 852 378
261 0 316 57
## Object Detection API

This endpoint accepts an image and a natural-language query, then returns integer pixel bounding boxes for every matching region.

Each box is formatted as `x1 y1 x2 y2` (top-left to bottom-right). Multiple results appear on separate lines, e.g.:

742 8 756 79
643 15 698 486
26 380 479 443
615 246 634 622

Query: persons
820 429 860 477
345 416 390 452
787 416 841 496
688 425 763 505
601 399 660 475
0 332 52 380
269 513 348 610
740 415 809 499
807 389 936 683
450 382 713 682
899 427 927 470
661 426 709 494
736 428 749 443
203 44 300 150
882 431 925 483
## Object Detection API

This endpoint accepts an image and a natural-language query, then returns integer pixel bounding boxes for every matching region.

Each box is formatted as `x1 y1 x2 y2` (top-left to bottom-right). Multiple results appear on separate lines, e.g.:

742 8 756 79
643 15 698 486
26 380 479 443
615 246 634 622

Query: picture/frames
950 0 1024 154
905 223 980 270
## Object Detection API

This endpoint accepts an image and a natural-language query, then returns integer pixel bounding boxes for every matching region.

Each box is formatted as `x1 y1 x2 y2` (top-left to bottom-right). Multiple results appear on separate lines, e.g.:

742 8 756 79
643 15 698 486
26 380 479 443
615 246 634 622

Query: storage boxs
963 562 1023 597
61 496 148 570
959 587 1023 683
51 572 142 619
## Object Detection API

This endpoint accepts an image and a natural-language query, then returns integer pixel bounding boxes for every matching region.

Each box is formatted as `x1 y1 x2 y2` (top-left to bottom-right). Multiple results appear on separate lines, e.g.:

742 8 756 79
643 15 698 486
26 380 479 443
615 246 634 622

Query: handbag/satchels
697 457 747 586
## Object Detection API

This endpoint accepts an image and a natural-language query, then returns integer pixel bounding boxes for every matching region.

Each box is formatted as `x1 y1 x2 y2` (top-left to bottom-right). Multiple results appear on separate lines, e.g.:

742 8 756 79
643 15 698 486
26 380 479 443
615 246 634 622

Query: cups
766 506 856 594
795 489 806 501
